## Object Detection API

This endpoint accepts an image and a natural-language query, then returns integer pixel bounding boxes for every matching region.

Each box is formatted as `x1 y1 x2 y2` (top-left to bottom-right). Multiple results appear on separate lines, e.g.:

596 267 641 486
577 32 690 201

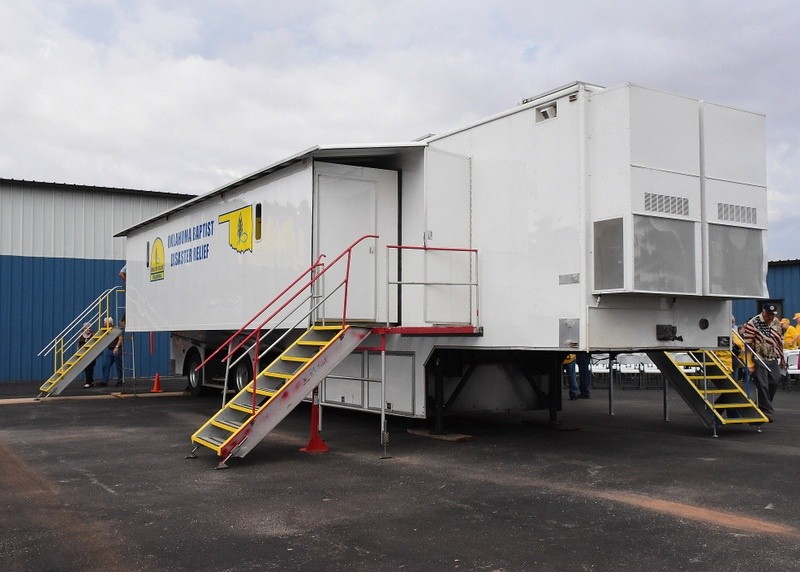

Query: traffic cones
150 373 165 393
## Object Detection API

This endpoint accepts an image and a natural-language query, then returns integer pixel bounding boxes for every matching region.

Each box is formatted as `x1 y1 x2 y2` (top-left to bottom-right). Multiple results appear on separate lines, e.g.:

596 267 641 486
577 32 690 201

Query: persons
712 304 800 422
563 353 592 400
76 317 123 388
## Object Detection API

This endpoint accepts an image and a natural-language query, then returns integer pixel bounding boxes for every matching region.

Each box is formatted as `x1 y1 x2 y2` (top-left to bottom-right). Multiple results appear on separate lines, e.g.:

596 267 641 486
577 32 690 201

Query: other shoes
114 381 120 387
767 416 773 423
119 320 124 327
96 381 107 387
83 383 93 387
738 378 743 383
569 393 580 400
579 393 590 399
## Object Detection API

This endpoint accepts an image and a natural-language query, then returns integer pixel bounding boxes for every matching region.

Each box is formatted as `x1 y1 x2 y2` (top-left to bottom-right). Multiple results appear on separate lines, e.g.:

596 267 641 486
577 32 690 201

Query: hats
780 318 790 324
793 313 800 319
763 303 778 315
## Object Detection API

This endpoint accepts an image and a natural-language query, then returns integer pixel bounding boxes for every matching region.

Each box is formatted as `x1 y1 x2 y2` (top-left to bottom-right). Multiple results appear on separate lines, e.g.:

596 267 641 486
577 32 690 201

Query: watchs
116 345 118 348
